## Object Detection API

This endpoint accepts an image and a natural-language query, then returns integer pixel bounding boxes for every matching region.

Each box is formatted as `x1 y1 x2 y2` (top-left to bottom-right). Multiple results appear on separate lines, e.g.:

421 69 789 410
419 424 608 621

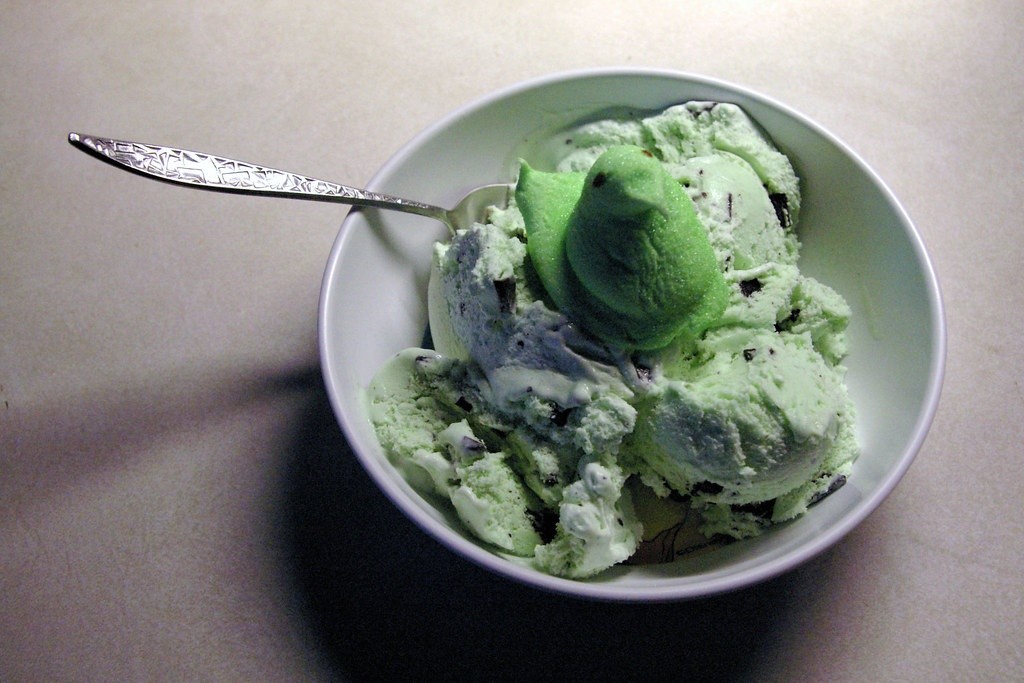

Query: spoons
67 131 513 235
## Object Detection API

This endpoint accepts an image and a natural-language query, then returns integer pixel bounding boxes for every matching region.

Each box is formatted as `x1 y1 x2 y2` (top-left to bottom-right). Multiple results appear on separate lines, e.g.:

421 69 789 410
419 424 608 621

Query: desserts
365 102 862 578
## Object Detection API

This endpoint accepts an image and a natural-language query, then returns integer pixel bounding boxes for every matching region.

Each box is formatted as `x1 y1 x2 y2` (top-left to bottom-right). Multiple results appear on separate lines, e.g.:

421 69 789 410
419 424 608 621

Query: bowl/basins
313 65 949 601
